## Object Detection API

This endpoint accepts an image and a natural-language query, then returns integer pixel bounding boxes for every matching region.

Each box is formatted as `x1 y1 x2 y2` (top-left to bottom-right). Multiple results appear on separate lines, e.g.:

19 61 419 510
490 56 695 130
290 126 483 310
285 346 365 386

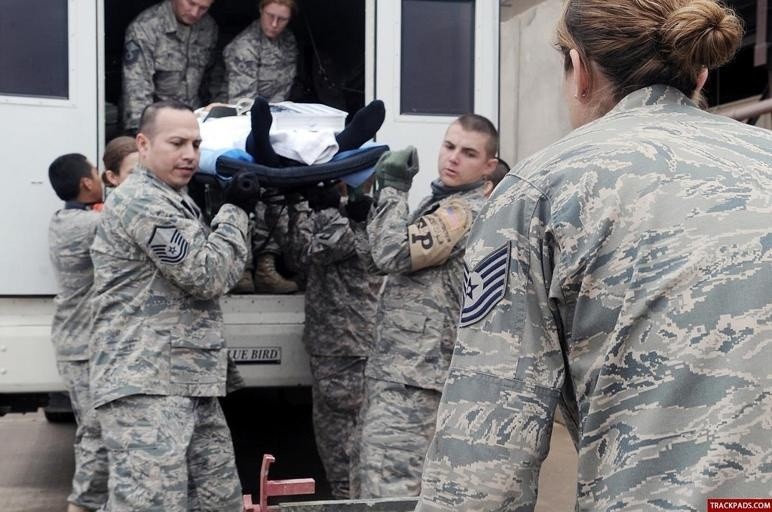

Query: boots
235 272 254 294
255 255 298 295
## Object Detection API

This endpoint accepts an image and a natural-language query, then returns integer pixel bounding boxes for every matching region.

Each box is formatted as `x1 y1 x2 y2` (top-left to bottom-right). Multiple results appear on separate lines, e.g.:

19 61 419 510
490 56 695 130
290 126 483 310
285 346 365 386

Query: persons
406 1 772 512
89 101 255 512
45 153 110 512
264 177 386 499
193 98 388 166
346 114 503 499
219 0 299 106
119 0 227 135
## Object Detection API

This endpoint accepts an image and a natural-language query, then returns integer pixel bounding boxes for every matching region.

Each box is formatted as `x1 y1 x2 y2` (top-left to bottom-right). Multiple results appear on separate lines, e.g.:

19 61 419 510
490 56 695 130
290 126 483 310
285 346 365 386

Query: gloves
309 183 372 222
226 169 261 212
375 143 420 193
